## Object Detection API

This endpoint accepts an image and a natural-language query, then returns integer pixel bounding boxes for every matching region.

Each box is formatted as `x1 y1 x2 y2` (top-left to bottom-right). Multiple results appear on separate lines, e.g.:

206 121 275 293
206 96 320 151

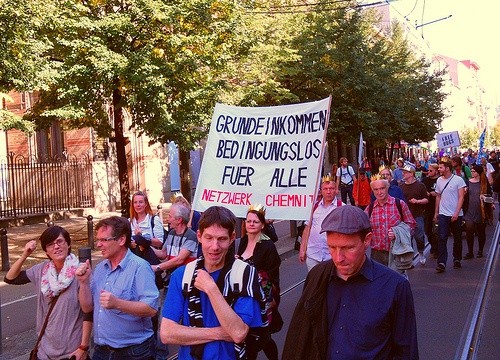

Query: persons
137 203 198 360
75 216 161 360
433 161 467 272
299 175 351 272
359 174 416 273
159 205 264 360
127 189 278 258
236 210 282 360
328 148 500 266
301 205 419 360
4 226 93 360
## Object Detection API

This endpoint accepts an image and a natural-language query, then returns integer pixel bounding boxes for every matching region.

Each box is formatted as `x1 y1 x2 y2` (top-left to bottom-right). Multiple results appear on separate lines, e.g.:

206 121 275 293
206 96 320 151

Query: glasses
94 236 120 245
428 169 432 172
47 239 65 248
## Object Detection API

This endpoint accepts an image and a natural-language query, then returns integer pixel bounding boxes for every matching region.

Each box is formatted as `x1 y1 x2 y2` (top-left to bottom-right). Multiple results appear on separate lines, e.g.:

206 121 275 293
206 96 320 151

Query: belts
97 343 139 353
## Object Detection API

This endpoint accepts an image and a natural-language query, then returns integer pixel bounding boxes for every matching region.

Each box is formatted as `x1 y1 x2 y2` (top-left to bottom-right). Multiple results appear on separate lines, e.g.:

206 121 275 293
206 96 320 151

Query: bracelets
78 344 90 352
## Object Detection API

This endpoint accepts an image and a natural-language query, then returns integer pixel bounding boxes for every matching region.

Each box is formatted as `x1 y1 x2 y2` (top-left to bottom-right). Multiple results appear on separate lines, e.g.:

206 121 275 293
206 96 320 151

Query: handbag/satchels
235 327 278 360
263 223 278 243
28 349 41 360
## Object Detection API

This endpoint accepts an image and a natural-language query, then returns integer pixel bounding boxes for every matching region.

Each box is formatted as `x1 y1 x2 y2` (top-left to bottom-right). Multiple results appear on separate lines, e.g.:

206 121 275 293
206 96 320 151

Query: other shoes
464 253 474 259
419 254 427 264
435 264 445 273
453 261 461 269
477 250 483 257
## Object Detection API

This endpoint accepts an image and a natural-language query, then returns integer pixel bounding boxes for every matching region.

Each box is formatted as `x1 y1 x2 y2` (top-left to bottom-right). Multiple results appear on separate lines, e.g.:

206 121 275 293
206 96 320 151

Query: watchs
156 264 160 272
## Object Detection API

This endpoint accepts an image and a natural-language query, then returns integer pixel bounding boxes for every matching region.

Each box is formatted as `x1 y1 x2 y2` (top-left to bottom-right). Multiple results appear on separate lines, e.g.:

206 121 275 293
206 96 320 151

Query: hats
319 205 371 234
399 165 416 174
470 165 483 175
